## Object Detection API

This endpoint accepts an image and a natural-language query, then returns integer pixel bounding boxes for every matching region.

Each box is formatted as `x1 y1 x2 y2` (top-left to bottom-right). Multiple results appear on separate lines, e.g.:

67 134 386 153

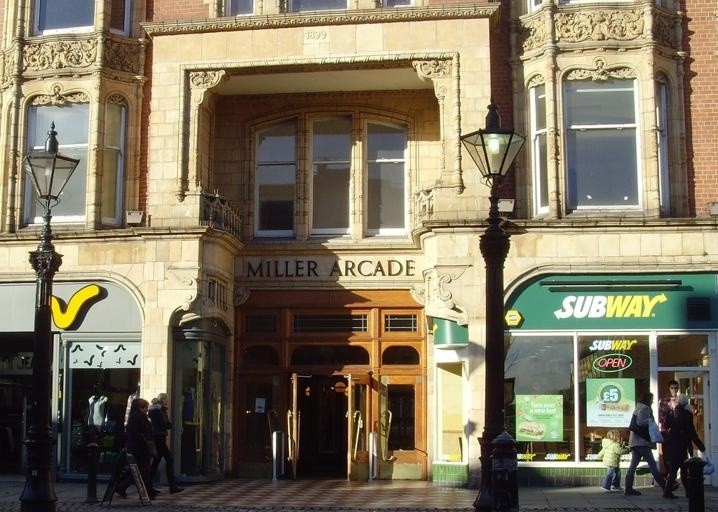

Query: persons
657 380 681 475
625 391 679 495
658 394 706 499
87 382 109 439
112 398 158 500
595 429 631 492
148 392 185 494
115 398 161 497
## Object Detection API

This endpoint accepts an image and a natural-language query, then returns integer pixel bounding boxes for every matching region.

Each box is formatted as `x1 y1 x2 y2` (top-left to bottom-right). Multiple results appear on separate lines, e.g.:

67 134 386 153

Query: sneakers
114 486 185 499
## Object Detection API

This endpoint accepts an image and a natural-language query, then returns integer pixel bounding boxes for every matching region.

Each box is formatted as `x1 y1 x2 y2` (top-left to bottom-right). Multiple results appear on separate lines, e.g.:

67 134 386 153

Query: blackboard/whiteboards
126 463 150 503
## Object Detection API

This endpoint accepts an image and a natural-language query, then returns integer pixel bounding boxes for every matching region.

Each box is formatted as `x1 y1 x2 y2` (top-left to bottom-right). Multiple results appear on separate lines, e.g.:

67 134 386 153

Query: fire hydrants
490 425 521 512
681 457 709 511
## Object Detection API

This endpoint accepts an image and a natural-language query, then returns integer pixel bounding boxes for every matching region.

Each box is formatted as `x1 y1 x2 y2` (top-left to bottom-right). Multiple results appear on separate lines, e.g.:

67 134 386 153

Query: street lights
18 120 82 512
460 98 528 511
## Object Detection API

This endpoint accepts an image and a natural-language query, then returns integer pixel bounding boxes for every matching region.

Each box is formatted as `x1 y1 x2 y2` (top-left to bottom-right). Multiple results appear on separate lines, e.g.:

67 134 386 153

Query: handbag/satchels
629 413 694 457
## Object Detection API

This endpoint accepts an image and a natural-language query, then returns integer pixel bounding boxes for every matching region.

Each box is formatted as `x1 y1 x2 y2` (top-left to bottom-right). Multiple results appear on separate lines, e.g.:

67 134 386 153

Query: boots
601 473 688 500
